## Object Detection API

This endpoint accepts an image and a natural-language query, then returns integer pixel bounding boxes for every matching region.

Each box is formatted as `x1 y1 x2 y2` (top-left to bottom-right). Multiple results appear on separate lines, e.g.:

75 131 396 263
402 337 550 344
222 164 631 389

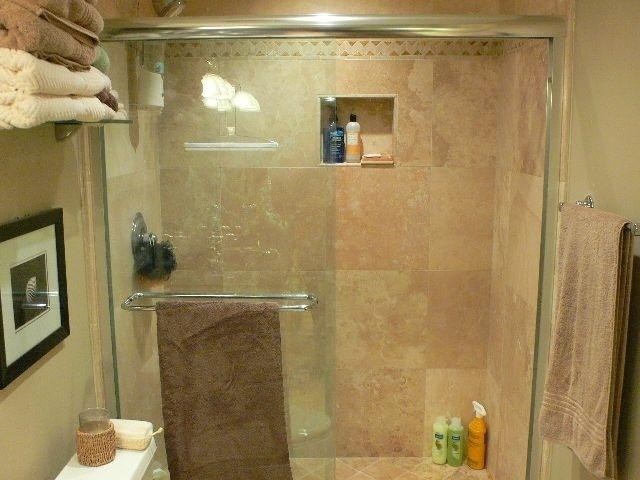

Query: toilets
140 351 333 459
52 439 159 480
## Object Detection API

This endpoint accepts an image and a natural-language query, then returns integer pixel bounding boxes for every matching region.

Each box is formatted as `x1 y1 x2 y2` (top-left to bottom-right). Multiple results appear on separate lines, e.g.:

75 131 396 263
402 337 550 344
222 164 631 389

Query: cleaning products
467 401 486 470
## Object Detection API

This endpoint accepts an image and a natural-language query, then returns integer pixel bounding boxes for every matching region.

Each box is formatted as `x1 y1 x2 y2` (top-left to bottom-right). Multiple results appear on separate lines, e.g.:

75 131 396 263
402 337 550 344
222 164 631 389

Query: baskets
75 420 117 467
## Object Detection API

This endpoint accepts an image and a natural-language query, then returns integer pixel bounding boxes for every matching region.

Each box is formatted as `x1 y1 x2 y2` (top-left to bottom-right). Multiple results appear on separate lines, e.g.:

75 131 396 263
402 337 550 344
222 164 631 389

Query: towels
0 0 126 130
535 198 633 478
154 303 293 480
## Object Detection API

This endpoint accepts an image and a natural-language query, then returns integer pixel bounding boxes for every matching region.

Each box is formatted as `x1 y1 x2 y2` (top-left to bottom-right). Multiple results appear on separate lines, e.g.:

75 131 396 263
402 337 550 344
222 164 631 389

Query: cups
78 406 111 434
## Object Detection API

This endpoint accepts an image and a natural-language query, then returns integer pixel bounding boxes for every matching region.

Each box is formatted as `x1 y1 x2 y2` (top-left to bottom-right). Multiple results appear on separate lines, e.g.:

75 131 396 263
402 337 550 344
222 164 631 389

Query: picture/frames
1 206 70 389
